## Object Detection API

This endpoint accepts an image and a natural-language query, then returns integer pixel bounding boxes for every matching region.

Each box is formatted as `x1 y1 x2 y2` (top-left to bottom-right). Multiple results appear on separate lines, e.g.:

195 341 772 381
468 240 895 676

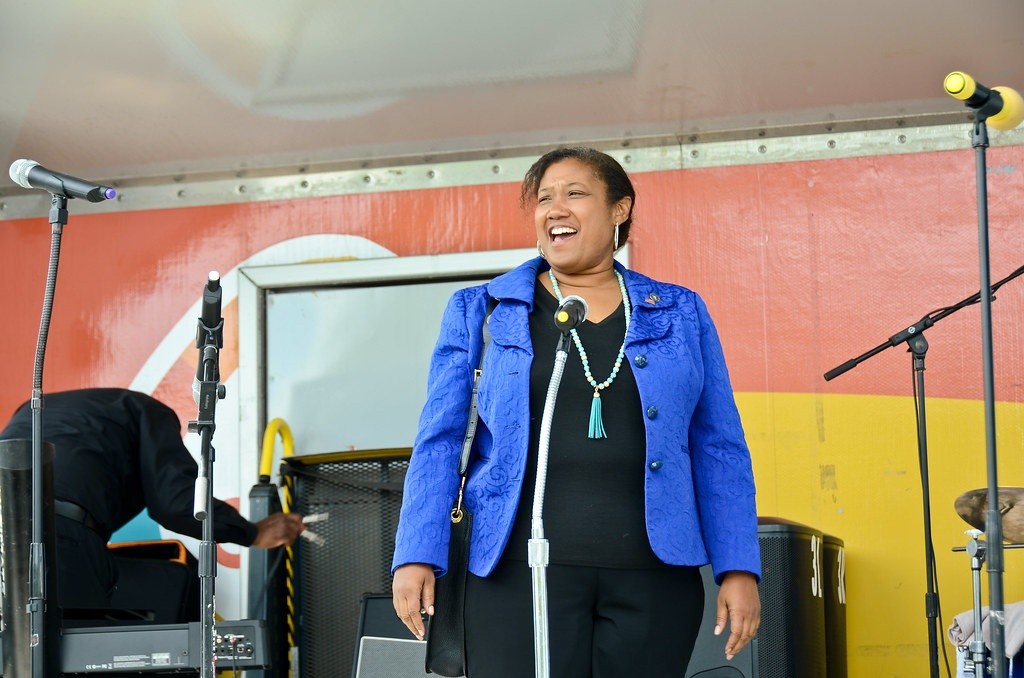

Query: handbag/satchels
425 502 472 677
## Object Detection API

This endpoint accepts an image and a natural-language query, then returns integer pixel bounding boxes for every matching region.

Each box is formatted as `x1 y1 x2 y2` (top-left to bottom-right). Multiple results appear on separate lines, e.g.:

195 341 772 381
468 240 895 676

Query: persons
1 388 304 677
390 147 763 678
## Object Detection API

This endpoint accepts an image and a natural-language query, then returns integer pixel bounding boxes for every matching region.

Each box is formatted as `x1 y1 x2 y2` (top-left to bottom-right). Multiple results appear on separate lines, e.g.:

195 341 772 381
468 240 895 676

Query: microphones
553 295 588 331
8 158 116 204
943 72 1024 131
191 271 226 406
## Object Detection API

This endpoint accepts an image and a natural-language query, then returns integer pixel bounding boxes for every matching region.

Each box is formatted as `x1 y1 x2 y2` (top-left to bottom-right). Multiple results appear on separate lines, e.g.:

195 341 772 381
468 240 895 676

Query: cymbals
952 484 1024 545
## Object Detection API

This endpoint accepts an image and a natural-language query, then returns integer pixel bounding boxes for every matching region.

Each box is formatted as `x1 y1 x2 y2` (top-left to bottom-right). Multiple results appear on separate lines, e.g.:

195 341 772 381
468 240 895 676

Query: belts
44 498 105 536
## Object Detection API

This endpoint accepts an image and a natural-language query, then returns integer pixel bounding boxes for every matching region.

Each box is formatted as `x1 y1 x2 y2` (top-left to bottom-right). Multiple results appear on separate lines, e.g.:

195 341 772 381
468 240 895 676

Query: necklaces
547 270 632 437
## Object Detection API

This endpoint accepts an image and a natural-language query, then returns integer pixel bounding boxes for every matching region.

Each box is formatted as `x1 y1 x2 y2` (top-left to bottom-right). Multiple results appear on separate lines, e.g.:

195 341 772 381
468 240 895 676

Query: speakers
683 516 848 678
